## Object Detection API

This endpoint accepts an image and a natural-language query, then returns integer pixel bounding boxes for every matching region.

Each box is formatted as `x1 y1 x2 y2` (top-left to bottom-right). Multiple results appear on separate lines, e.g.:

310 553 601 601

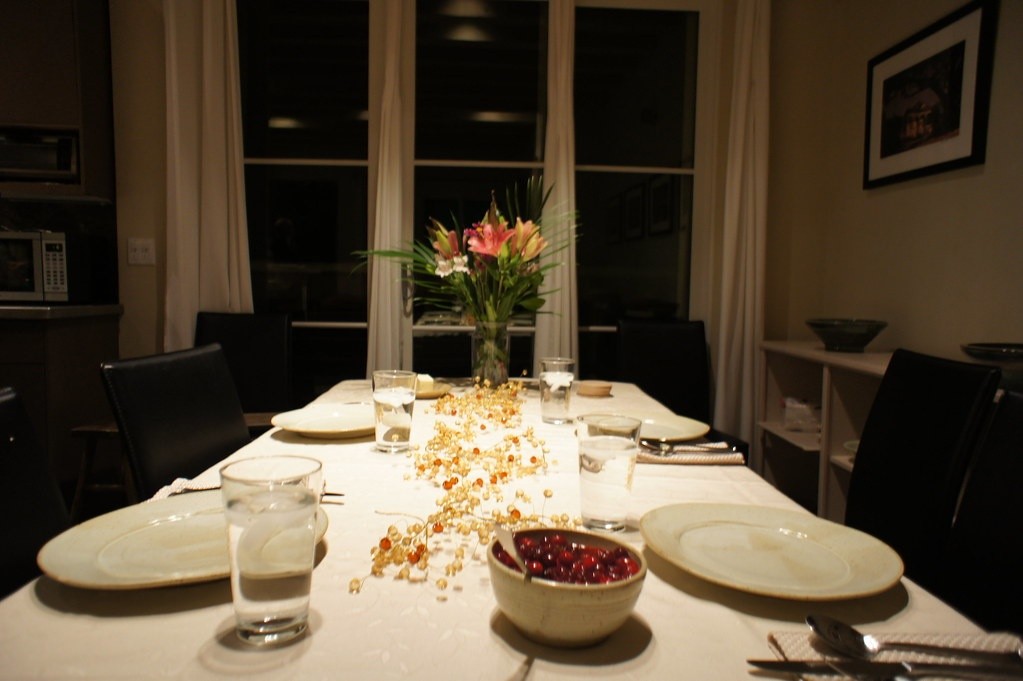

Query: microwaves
0 225 68 306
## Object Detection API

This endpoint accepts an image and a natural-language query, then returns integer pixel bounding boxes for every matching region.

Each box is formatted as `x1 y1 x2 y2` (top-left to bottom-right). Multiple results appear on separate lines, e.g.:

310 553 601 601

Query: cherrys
498 533 637 584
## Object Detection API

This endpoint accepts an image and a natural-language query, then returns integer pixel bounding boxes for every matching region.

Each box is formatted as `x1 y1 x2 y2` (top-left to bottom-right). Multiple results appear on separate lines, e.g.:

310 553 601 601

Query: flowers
347 174 578 379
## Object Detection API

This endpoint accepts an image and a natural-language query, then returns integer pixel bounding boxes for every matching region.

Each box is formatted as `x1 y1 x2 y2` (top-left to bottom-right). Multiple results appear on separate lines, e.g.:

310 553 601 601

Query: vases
473 317 512 387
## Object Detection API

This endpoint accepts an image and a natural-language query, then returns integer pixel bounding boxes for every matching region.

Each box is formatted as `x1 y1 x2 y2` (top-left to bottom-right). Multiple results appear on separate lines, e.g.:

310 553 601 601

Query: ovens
0 128 78 184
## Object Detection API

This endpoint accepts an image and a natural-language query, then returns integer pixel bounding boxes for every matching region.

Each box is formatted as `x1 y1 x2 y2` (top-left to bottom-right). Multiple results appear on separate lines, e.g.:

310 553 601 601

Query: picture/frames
862 0 1000 191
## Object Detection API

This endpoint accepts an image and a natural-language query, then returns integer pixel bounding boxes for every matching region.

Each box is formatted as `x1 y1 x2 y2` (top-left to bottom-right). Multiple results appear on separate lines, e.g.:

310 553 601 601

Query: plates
271 404 377 438
38 490 328 591
417 383 450 398
638 502 904 600
635 416 711 445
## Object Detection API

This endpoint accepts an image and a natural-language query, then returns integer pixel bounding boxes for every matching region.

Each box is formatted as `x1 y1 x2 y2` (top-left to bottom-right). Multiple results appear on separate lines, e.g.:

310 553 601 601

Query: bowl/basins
806 318 887 354
576 380 611 396
843 440 859 461
577 413 642 439
488 528 647 649
961 342 1023 391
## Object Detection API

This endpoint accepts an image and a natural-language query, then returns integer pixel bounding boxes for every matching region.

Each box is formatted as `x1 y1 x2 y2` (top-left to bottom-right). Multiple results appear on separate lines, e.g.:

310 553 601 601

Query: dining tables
0 380 1023 681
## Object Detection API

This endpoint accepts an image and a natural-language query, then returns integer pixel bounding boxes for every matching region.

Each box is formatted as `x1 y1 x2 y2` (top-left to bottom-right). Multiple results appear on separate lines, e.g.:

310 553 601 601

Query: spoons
805 614 1023 669
640 439 736 452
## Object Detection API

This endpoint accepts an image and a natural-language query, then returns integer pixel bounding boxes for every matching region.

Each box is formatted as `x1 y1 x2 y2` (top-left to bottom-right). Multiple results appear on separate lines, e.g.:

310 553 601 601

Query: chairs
597 319 751 468
841 347 1003 607
1 310 295 599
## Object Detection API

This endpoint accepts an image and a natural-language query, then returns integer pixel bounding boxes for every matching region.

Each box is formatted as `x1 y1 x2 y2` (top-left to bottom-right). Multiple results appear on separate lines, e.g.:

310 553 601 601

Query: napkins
153 477 220 494
633 441 744 465
765 631 1023 681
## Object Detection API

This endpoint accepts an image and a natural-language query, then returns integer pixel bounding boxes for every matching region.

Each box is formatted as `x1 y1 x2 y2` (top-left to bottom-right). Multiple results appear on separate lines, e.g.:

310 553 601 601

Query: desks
0 306 127 573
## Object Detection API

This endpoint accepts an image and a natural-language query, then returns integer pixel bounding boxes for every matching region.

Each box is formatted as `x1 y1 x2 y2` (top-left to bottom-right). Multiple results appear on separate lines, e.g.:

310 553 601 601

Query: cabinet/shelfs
760 341 908 527
0 0 84 195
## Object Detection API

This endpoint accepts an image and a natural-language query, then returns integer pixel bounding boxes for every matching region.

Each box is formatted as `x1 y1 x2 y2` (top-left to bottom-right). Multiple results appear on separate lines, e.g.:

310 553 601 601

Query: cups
372 369 416 454
219 454 323 647
538 357 574 425
578 426 638 533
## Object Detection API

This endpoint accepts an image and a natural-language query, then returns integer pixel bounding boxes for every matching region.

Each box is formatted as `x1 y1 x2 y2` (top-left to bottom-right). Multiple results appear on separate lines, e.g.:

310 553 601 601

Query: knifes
747 658 1023 678
